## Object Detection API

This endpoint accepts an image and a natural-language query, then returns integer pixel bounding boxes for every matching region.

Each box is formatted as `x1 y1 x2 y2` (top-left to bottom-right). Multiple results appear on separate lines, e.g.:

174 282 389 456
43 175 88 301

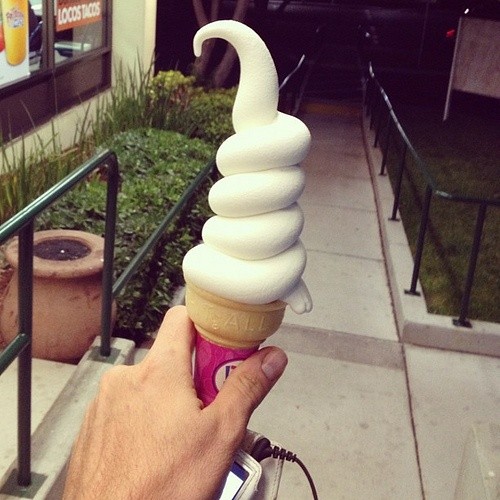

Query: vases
0 228 119 365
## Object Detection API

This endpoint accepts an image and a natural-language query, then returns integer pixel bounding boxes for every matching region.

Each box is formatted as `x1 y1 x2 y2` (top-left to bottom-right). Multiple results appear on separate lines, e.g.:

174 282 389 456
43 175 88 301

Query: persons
56 305 289 500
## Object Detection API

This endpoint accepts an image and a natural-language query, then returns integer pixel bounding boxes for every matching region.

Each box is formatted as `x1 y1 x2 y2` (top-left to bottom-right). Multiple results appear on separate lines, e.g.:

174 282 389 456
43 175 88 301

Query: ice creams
181 19 314 411
